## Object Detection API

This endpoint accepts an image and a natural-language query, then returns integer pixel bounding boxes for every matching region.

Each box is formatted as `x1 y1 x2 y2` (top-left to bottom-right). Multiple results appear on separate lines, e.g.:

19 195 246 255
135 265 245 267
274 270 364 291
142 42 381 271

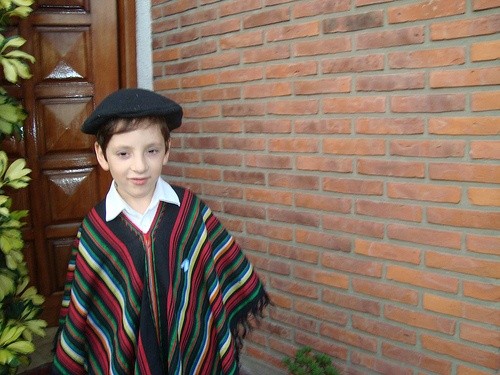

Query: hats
80 88 186 133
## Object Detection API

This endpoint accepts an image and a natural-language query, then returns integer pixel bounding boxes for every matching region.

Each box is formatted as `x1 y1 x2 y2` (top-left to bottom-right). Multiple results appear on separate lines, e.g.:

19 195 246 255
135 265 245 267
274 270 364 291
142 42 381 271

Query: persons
49 88 271 375
